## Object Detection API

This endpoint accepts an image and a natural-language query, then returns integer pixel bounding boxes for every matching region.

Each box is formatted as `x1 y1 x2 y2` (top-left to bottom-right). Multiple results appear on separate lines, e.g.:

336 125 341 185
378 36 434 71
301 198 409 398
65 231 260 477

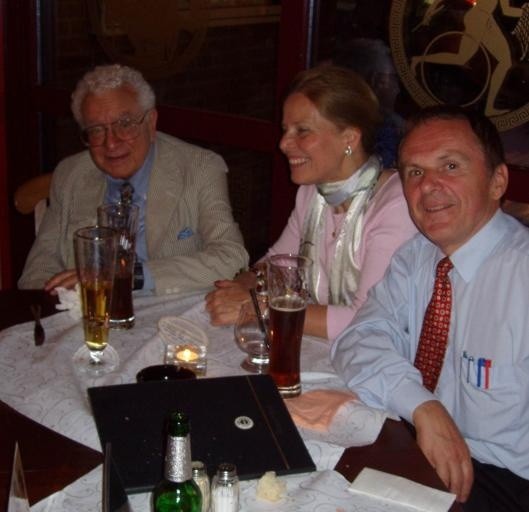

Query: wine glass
234 298 271 370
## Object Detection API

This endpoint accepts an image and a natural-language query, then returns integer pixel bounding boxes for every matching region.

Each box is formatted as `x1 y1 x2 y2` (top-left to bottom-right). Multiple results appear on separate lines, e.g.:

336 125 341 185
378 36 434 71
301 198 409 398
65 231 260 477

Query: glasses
83 114 146 146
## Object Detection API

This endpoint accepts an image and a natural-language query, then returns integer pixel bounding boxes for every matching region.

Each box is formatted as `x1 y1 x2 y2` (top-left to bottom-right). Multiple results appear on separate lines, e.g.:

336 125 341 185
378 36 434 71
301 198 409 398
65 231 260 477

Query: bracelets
131 261 146 291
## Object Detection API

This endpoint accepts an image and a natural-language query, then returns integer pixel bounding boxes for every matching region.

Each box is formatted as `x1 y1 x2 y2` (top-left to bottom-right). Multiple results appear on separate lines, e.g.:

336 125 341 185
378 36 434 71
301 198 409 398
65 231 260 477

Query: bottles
146 408 203 511
190 462 240 512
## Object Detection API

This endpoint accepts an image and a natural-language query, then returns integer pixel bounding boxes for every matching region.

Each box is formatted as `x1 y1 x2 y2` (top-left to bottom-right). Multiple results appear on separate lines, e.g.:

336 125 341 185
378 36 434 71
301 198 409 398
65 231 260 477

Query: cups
169 342 208 379
95 200 142 327
72 222 123 372
262 252 315 399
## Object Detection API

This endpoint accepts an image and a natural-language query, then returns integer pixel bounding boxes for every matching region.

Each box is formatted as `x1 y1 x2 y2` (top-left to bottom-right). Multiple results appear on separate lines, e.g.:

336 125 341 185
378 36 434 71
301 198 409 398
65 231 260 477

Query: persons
200 63 423 345
329 106 528 511
18 62 254 305
409 0 529 117
325 33 407 171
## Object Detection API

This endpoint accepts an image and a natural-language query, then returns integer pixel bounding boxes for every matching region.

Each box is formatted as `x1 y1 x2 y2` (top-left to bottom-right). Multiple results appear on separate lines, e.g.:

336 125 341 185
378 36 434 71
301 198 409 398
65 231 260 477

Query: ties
118 184 134 204
414 257 453 394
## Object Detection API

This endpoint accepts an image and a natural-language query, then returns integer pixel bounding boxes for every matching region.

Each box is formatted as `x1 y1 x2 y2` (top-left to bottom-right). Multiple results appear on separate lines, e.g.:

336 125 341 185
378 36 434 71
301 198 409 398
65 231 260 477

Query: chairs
16 169 55 240
503 168 529 206
